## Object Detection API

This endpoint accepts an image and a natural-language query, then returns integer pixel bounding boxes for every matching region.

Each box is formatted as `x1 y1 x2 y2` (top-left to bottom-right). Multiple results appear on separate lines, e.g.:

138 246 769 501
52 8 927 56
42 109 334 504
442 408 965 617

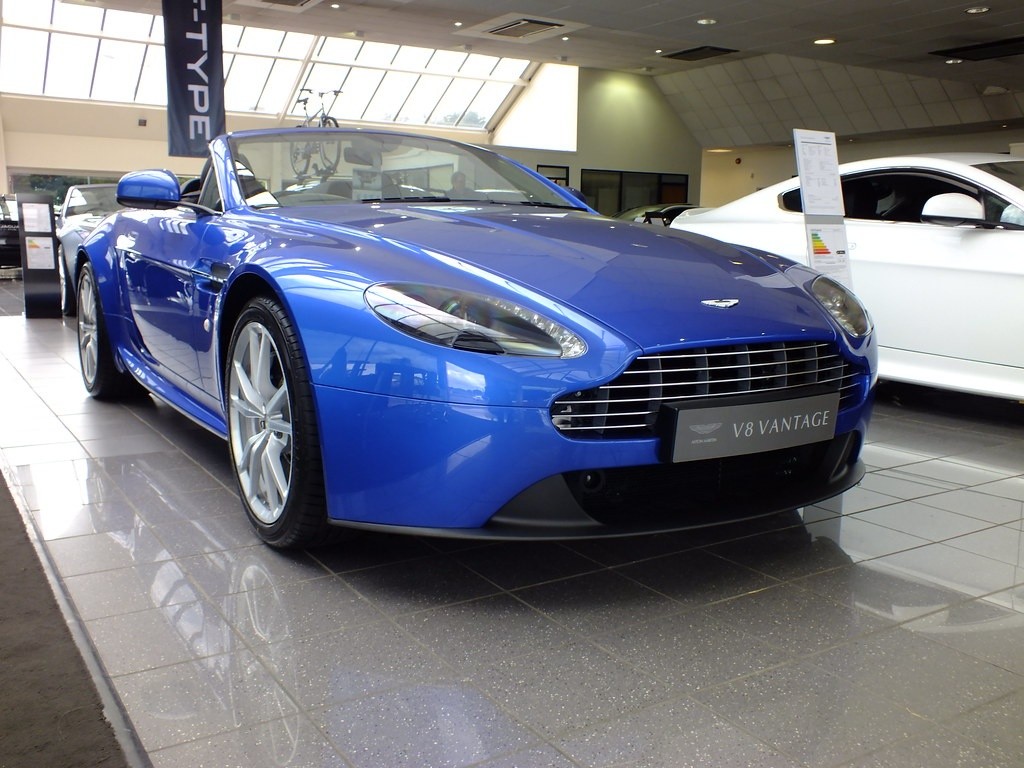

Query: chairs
201 154 257 210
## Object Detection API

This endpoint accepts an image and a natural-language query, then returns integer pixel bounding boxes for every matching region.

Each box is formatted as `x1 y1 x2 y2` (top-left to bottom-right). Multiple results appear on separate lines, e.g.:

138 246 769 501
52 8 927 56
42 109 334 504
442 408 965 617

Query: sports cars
54 181 131 317
73 127 879 554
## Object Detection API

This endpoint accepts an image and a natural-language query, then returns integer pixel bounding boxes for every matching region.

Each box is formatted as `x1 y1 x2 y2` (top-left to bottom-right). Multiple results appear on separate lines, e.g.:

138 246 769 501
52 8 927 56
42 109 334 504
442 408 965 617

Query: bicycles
290 88 343 175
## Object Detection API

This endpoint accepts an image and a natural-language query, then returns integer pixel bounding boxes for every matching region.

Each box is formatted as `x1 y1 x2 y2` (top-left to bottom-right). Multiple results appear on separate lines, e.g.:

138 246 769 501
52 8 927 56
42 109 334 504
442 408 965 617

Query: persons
444 172 475 198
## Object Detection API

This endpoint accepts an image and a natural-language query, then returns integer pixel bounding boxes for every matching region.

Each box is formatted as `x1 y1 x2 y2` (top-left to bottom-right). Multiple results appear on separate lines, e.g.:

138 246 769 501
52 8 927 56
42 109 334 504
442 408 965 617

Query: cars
609 149 1024 405
